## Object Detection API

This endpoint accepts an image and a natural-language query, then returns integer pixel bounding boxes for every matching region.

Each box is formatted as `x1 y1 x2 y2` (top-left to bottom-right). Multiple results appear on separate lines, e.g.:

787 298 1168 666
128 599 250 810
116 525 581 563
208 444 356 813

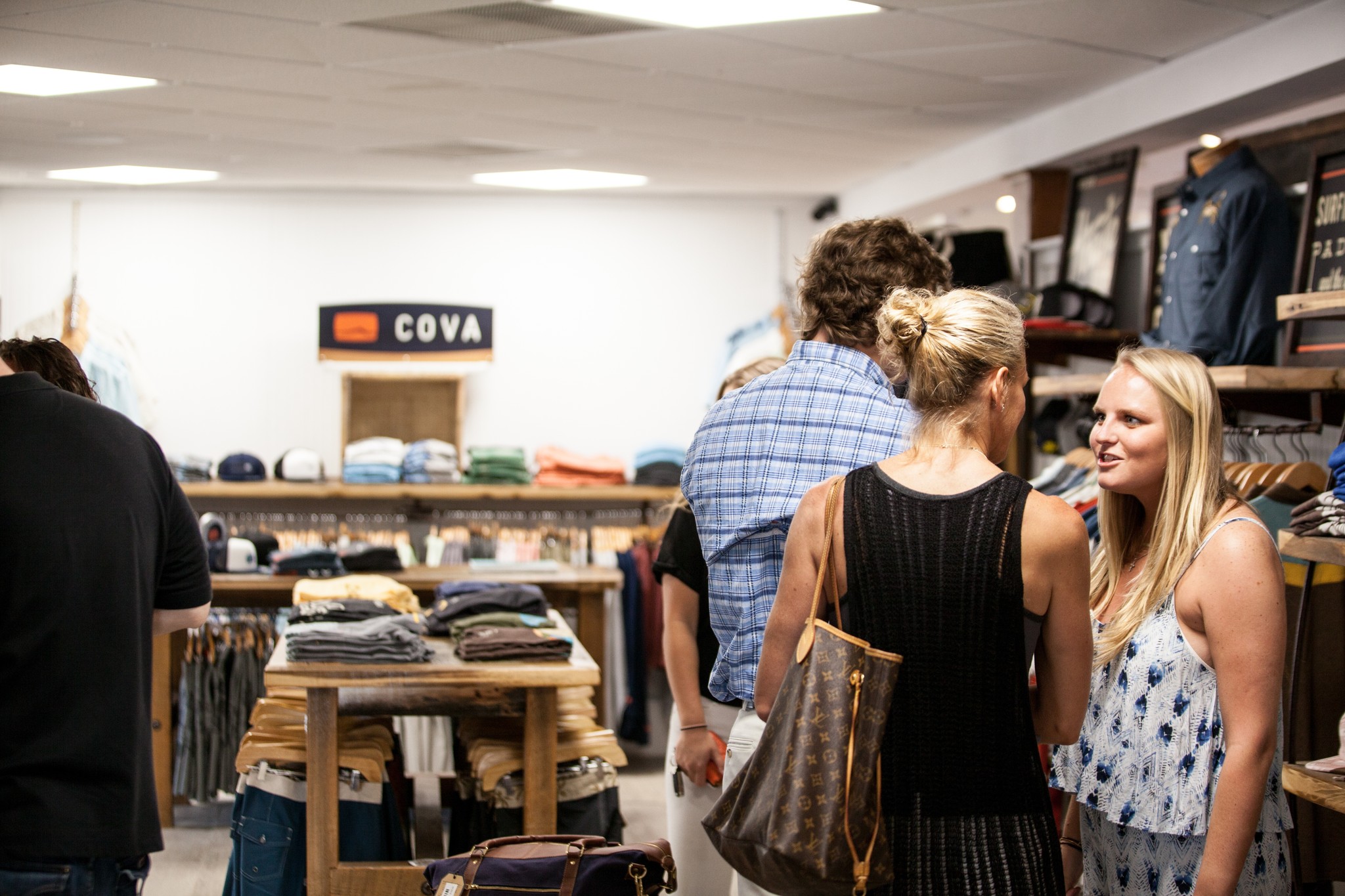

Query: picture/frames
1143 175 1188 332
1059 144 1140 319
1280 132 1344 366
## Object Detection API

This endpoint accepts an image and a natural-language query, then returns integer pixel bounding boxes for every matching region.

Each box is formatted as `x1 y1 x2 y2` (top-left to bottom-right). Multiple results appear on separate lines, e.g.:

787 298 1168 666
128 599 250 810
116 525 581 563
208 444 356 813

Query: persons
0 354 213 896
1046 345 1296 896
1163 138 1281 365
0 336 97 402
755 285 1093 896
679 216 954 896
651 357 789 896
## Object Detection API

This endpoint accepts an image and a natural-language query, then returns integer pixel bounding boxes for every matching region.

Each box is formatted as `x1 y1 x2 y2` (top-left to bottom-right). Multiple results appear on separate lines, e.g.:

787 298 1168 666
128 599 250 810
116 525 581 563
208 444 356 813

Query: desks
149 564 626 830
262 600 602 896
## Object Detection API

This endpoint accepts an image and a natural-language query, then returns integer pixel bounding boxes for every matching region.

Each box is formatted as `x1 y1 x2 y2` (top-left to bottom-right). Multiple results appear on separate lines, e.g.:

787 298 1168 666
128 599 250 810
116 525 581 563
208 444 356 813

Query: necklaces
1129 524 1148 572
912 443 988 460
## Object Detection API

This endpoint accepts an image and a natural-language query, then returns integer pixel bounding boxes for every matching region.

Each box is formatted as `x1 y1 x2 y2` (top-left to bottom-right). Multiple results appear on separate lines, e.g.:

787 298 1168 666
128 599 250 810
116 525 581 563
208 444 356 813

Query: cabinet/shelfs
1267 515 1345 896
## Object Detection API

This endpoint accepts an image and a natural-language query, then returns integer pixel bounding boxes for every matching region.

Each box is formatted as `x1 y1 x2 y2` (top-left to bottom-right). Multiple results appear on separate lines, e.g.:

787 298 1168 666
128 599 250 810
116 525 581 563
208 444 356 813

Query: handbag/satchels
422 834 677 896
700 475 904 896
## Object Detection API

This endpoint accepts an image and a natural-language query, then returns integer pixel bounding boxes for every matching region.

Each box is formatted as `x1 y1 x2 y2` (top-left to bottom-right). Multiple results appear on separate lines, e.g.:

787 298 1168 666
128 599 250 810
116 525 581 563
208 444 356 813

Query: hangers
1061 406 1330 496
186 506 670 793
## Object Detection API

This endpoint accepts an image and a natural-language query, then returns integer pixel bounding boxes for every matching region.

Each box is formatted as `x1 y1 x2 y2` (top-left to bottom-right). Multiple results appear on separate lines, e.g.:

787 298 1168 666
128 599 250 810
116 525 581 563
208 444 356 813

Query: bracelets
680 724 707 731
1059 837 1083 852
